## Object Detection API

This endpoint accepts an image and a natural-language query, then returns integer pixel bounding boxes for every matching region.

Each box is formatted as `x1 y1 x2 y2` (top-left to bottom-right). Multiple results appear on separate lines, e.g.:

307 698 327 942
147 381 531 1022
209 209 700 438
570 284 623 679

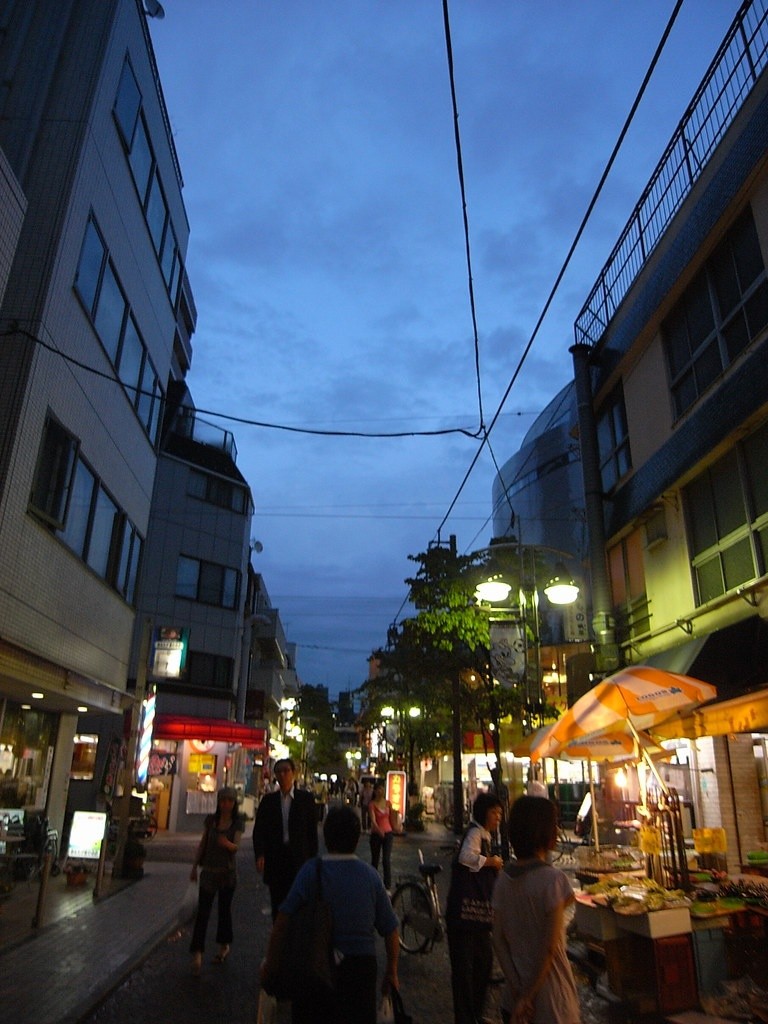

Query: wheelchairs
15 814 60 882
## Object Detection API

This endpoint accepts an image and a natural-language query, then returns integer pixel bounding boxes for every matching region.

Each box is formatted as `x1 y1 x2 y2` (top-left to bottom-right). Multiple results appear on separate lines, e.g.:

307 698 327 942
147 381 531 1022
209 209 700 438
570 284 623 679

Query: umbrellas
510 665 717 850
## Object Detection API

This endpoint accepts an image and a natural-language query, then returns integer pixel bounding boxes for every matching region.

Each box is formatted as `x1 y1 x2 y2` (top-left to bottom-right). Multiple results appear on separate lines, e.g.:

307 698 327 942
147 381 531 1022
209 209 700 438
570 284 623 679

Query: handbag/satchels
391 984 412 1024
389 806 404 833
459 825 495 924
378 994 394 1024
257 989 277 1024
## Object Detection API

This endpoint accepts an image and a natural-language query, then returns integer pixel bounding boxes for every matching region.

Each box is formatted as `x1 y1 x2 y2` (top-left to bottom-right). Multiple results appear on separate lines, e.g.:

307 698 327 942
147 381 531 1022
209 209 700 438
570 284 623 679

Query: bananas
583 876 684 911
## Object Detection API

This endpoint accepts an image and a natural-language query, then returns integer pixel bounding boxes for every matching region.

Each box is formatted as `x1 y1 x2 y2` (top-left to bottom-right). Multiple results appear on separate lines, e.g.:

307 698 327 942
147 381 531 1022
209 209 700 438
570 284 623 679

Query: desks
690 897 768 918
559 862 646 884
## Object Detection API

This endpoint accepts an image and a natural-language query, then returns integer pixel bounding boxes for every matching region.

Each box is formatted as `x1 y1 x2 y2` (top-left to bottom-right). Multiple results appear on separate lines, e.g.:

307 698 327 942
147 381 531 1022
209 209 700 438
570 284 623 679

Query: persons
186 760 580 1024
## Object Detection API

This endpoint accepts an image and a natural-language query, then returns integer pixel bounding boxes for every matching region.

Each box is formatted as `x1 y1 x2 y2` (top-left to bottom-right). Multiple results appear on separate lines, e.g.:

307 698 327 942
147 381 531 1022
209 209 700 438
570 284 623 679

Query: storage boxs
575 887 730 1016
694 828 727 872
731 912 768 991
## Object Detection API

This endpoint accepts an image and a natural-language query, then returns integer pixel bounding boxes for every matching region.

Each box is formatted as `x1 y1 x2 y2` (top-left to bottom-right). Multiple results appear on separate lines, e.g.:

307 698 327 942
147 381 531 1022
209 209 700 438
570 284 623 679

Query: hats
476 792 504 807
218 788 237 802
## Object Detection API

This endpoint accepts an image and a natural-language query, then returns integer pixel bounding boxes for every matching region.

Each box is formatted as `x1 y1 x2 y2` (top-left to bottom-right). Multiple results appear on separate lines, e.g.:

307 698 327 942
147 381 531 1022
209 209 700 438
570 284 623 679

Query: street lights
468 542 582 784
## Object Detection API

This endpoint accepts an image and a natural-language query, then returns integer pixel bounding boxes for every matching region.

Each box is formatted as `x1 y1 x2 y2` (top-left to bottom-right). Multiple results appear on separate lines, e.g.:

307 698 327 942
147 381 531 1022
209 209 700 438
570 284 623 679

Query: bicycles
386 863 452 960
443 807 471 834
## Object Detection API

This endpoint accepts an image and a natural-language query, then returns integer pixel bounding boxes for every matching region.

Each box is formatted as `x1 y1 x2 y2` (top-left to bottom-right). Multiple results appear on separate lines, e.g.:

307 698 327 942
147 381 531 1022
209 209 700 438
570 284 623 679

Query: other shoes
219 948 230 960
386 890 391 896
362 830 366 834
191 958 202 976
367 829 371 834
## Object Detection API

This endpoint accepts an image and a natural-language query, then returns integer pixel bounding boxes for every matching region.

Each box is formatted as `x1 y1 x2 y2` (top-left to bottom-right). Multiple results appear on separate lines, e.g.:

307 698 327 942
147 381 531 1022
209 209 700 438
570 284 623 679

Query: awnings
127 708 267 746
641 613 767 705
